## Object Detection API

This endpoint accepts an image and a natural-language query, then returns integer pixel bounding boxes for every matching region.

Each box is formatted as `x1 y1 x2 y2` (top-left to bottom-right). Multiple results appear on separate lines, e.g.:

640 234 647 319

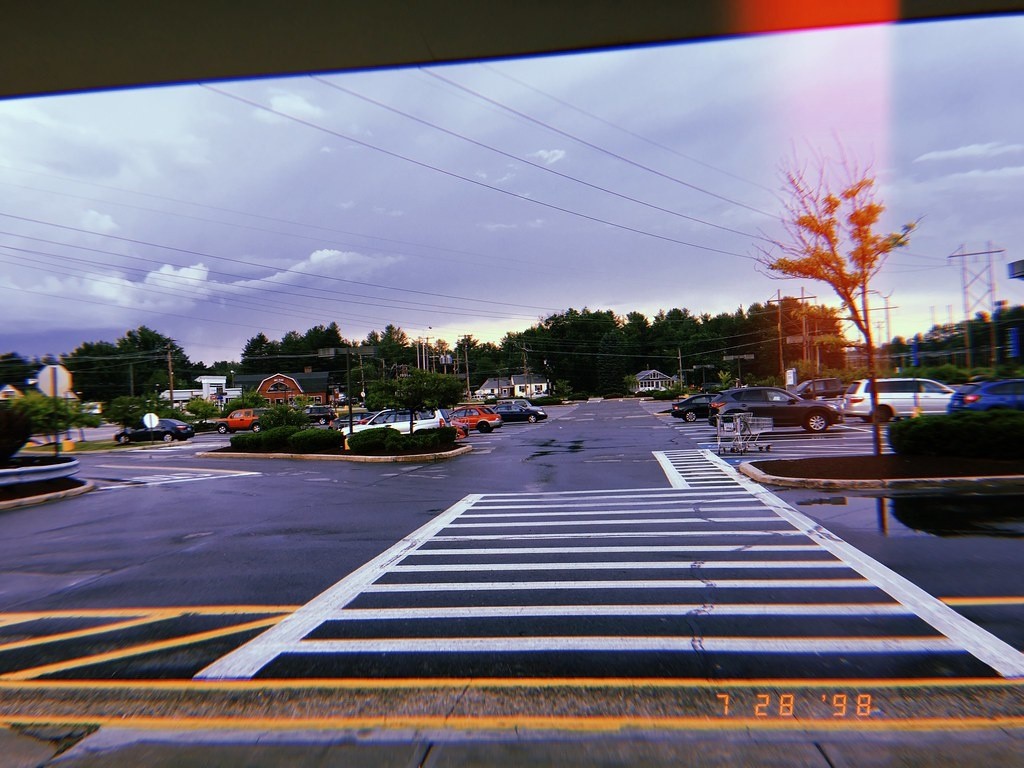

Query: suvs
946 376 1024 415
707 388 845 434
341 406 449 439
838 377 956 424
217 407 271 434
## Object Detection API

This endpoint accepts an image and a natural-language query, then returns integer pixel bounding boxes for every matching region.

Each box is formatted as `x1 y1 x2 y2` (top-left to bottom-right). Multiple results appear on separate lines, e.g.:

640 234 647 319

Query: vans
495 399 544 412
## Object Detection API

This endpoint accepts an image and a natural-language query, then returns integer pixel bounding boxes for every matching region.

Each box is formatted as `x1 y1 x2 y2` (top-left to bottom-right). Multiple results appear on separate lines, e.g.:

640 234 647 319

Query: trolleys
715 410 774 457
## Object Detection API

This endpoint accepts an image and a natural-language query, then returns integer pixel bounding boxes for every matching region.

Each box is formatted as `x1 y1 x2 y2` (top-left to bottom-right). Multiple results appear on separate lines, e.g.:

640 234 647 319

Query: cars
670 394 718 423
328 412 375 432
492 404 548 423
448 405 505 433
112 418 196 446
449 416 469 440
794 377 846 401
299 404 336 426
